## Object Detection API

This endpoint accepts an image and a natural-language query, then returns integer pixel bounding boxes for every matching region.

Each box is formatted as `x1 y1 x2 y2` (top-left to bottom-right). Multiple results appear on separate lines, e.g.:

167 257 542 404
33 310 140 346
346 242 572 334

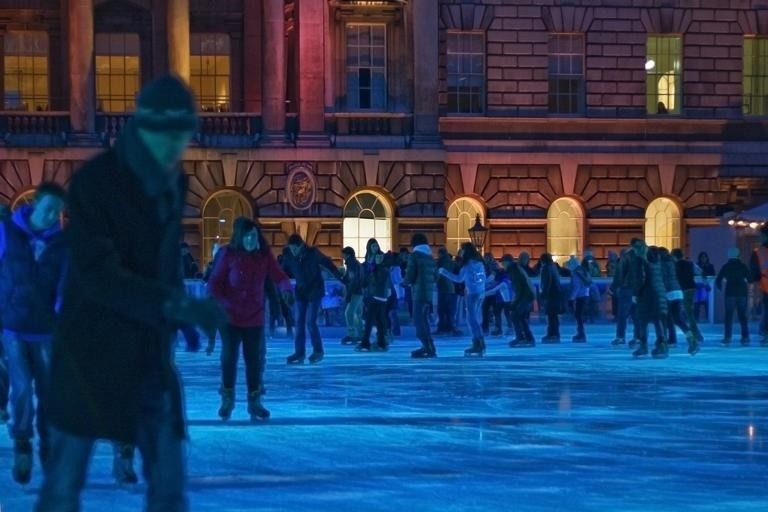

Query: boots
12 438 33 484
246 391 270 417
519 330 534 344
633 344 648 355
688 336 697 352
510 331 523 345
464 339 482 353
112 441 139 484
652 343 665 354
217 387 236 417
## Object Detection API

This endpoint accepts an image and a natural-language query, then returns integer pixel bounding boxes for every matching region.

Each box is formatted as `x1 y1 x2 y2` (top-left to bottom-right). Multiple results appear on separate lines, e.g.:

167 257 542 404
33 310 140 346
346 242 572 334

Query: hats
727 248 740 257
133 74 205 130
499 254 514 262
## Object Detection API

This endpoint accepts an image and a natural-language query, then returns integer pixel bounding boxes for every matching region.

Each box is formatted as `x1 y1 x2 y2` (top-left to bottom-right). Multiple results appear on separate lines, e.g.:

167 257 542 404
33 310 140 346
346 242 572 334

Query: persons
267 232 768 359
215 215 295 423
0 181 68 484
177 245 225 355
111 437 142 489
201 218 284 400
0 204 14 422
38 69 232 510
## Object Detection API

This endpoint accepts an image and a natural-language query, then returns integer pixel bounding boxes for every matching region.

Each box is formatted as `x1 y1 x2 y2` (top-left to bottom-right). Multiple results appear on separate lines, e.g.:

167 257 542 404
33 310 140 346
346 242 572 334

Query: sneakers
309 352 324 362
287 352 306 361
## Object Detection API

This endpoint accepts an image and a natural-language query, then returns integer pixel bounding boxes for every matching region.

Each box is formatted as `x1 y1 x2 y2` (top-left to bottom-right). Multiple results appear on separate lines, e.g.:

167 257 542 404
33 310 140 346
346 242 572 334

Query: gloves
282 291 294 306
